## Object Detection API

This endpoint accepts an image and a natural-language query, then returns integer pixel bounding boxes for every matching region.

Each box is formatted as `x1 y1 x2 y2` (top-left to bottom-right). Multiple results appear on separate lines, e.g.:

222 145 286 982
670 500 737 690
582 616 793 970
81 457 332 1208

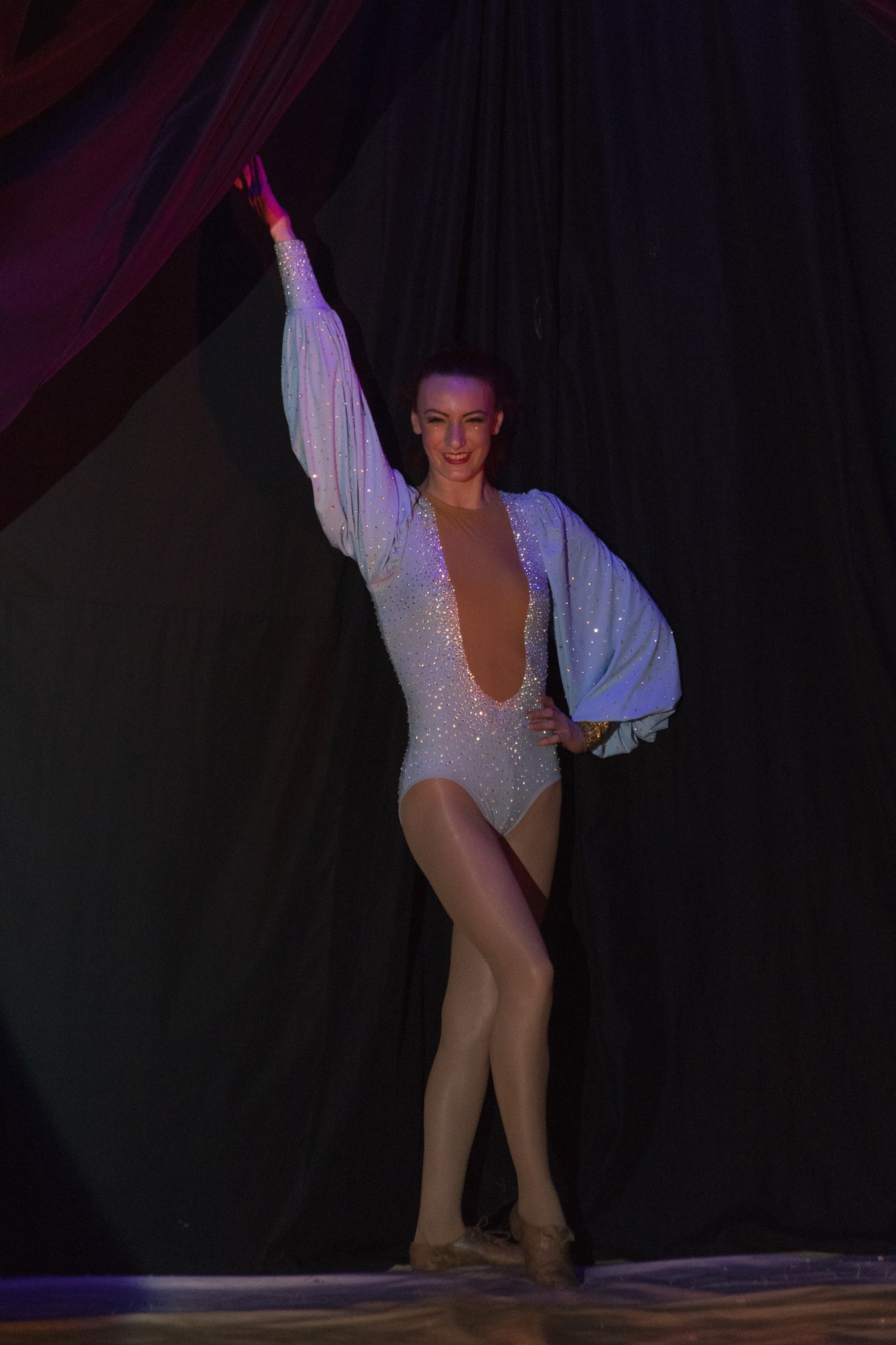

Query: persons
236 151 681 1285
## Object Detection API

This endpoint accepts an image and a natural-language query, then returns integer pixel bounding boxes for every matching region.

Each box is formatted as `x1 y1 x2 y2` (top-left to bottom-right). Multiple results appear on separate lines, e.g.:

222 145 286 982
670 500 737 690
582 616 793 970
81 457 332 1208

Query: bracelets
577 720 610 748
